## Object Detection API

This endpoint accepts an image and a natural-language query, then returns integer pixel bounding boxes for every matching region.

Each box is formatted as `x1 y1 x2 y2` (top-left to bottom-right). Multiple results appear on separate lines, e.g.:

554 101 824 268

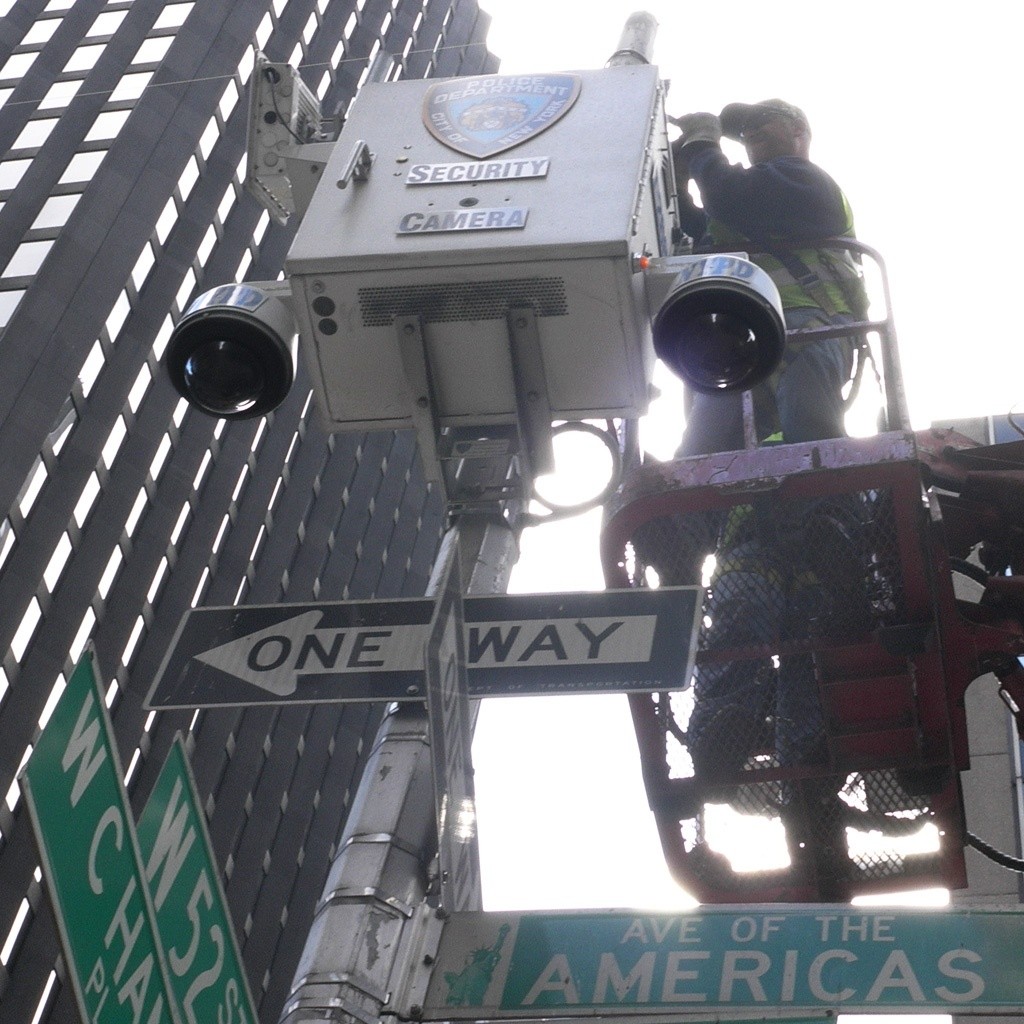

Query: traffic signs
144 585 704 709
15 635 183 1024
422 910 1024 1017
131 728 257 1024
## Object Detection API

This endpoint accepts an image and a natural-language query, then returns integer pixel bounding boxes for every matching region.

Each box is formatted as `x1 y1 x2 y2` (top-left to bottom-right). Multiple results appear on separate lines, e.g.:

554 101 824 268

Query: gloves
677 111 722 148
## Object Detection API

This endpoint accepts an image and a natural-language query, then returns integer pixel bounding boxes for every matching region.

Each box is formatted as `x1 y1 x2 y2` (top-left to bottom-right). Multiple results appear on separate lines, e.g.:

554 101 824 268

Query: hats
719 98 813 143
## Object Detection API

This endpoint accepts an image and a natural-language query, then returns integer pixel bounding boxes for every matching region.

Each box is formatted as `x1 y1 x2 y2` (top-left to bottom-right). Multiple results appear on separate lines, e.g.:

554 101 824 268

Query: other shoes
694 704 754 803
672 507 719 555
802 517 876 600
784 727 849 805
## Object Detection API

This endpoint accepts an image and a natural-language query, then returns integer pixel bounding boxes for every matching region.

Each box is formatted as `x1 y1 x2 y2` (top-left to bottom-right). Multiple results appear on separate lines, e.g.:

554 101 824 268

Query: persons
639 98 871 563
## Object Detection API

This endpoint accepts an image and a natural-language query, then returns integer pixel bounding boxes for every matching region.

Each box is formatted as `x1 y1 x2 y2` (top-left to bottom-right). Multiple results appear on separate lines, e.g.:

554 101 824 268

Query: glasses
737 112 794 144
